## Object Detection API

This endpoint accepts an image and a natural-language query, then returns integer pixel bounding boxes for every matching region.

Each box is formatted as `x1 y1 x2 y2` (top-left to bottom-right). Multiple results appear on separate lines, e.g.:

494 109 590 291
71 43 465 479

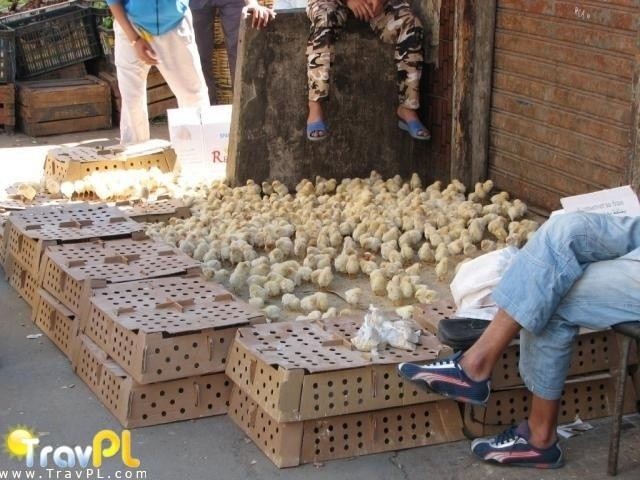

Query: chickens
15 166 194 204
146 171 541 322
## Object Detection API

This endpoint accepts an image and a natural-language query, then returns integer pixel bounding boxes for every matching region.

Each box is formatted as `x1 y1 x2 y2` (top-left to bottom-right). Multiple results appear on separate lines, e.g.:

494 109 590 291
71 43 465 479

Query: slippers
306 121 327 141
398 118 431 140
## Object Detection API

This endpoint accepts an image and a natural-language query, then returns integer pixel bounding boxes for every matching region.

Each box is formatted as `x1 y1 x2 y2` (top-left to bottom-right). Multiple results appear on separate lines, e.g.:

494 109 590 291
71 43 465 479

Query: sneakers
470 420 564 469
396 350 491 406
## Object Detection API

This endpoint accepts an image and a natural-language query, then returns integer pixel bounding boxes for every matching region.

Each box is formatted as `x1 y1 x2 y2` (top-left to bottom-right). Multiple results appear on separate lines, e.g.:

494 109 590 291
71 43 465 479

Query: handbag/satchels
437 318 490 349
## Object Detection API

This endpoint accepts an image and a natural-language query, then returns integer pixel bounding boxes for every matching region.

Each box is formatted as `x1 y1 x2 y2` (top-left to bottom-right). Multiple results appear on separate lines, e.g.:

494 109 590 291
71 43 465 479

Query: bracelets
131 35 141 47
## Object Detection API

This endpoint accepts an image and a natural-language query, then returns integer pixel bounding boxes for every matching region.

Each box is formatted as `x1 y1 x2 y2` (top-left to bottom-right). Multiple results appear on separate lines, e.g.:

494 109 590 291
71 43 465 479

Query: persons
106 0 210 147
189 1 276 106
397 211 640 468
304 0 432 142
